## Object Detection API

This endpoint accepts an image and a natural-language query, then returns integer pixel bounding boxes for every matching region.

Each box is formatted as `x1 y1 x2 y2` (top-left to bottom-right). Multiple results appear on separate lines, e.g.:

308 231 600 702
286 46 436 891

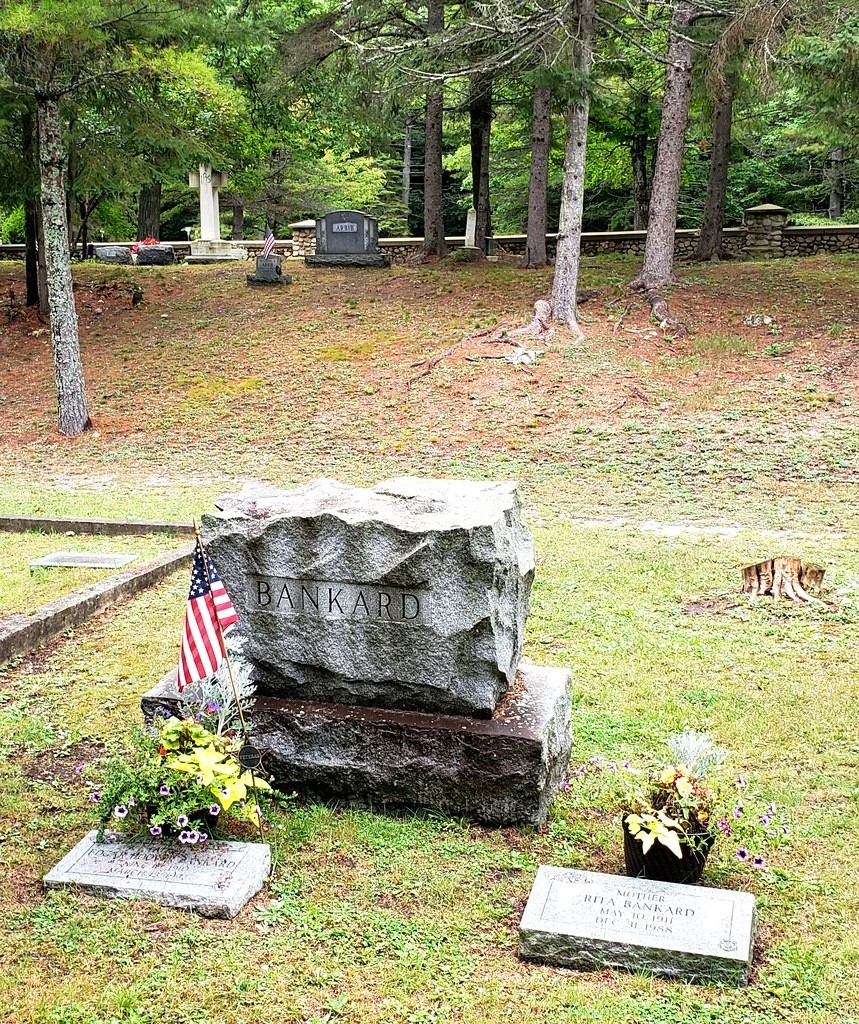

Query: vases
147 803 218 837
622 814 715 887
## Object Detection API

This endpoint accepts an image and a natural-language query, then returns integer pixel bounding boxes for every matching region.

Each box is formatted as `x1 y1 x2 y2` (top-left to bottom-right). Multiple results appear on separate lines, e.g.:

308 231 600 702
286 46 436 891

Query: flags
177 537 238 692
263 224 276 260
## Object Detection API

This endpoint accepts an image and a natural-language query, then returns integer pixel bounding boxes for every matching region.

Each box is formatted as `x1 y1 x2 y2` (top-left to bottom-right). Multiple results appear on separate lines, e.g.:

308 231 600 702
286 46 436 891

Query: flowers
558 755 789 867
75 634 299 852
